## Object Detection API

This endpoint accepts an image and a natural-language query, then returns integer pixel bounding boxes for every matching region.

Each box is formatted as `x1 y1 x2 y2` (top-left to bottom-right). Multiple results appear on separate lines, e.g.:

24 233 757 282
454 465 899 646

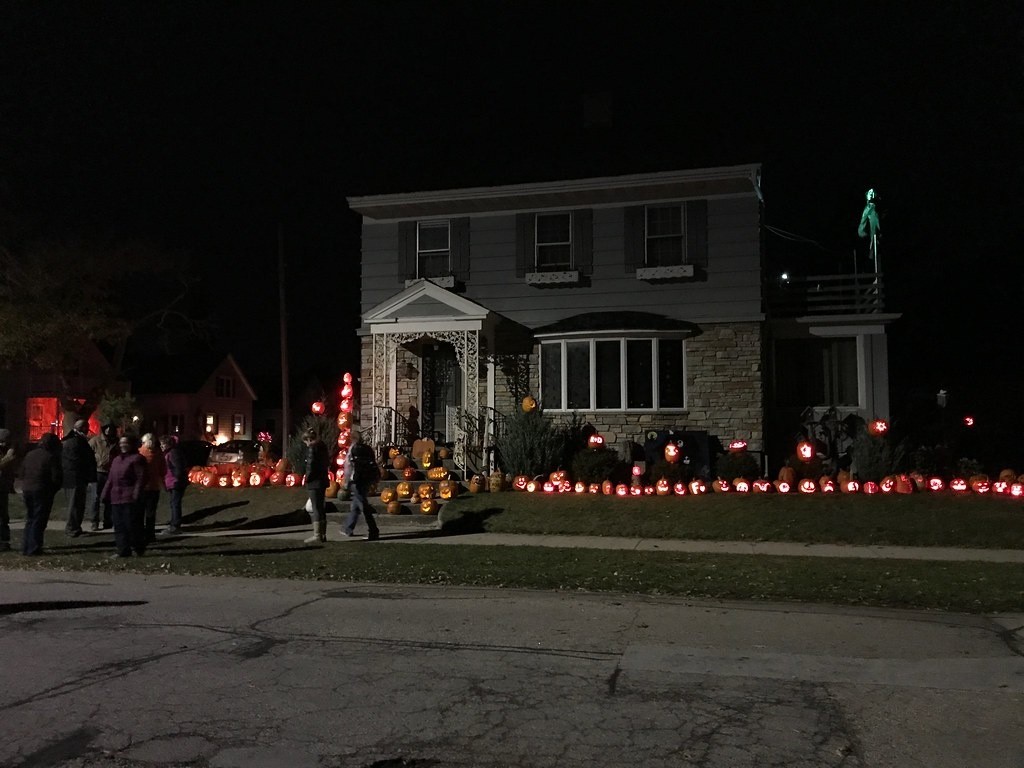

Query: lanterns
188 458 307 489
664 444 680 464
379 447 459 515
522 397 537 412
325 372 354 501
470 464 1024 498
311 402 325 414
868 419 888 436
728 440 747 453
588 434 604 449
797 442 815 464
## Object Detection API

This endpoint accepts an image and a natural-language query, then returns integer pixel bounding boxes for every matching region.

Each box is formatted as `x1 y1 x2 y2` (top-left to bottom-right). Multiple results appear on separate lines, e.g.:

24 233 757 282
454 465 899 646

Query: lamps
399 359 409 376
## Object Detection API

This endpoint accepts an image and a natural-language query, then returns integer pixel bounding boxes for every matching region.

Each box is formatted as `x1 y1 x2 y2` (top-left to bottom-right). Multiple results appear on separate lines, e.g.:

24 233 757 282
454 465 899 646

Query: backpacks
352 445 380 481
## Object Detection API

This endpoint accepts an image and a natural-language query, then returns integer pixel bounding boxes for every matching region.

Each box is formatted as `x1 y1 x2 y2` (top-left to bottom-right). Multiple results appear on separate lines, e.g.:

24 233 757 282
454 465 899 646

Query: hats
0 429 10 441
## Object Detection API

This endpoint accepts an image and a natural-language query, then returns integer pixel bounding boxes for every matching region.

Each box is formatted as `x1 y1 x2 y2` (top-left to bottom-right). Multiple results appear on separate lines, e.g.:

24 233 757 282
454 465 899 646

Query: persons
0 417 187 558
302 428 328 542
339 431 380 540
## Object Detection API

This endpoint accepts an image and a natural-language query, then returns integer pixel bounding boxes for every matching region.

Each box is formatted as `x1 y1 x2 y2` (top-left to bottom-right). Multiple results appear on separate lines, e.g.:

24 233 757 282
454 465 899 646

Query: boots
304 520 327 543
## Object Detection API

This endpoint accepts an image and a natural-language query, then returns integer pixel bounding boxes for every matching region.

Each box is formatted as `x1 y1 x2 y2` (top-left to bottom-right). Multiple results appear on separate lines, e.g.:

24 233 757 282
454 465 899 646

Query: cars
182 439 214 467
209 439 278 466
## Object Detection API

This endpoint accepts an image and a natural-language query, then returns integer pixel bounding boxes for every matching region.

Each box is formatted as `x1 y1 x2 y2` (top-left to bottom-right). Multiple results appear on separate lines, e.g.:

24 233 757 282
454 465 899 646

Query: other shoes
91 522 98 531
0 543 10 552
162 525 182 536
67 531 90 538
111 553 123 559
361 535 379 540
340 531 353 537
23 550 48 556
